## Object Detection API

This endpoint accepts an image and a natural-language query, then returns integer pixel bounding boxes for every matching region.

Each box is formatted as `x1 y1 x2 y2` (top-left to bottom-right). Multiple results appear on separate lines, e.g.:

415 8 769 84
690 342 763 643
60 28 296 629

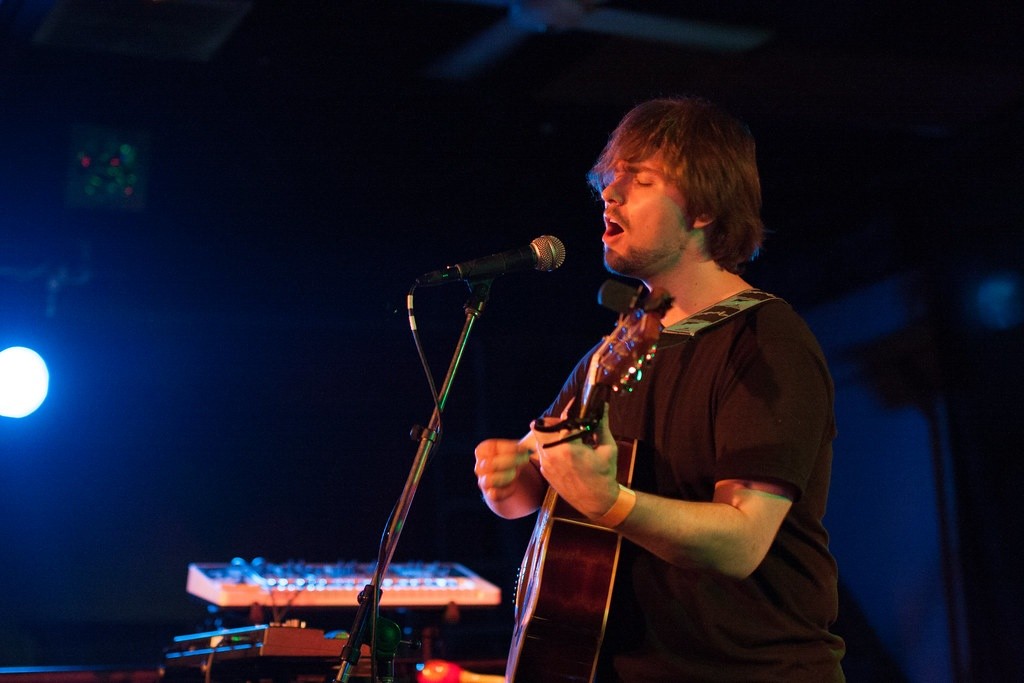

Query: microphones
417 235 565 287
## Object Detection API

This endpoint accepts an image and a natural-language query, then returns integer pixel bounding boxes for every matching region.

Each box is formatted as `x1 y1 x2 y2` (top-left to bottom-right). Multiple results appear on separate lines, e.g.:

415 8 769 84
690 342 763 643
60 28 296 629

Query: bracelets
594 483 637 528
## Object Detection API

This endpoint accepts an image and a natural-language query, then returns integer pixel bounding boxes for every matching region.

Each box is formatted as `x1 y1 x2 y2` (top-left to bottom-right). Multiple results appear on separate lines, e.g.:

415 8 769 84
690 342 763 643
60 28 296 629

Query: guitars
505 285 673 683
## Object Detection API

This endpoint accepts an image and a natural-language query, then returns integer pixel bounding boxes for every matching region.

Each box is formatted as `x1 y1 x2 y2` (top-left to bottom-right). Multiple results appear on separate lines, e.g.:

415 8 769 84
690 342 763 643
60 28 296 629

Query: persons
474 97 849 683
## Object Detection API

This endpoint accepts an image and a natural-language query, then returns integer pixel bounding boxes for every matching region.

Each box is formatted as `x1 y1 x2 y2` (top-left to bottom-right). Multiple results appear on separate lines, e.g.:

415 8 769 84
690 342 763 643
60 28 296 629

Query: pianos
189 556 502 612
